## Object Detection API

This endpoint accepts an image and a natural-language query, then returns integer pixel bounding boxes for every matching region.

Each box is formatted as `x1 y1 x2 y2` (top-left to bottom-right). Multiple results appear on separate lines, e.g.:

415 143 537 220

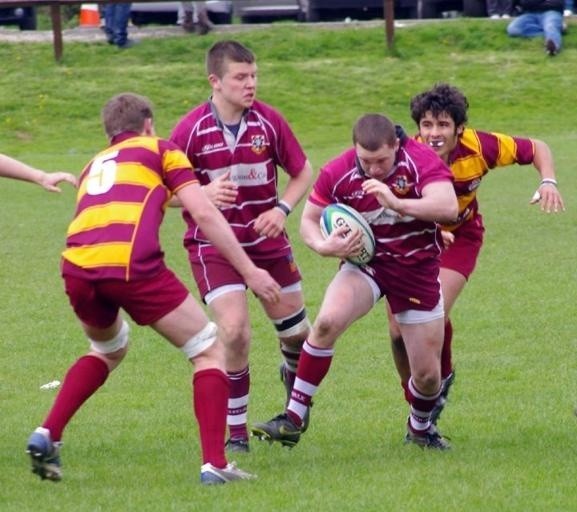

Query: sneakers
200 462 255 485
225 365 313 452
407 430 451 449
25 427 63 480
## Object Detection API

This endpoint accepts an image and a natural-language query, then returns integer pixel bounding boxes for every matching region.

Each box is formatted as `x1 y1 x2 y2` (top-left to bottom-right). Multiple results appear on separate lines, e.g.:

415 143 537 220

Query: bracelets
539 178 557 186
272 200 290 216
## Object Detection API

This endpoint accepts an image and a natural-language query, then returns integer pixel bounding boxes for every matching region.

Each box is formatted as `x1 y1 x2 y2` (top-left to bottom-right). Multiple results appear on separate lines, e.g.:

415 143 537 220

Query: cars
0 6 36 29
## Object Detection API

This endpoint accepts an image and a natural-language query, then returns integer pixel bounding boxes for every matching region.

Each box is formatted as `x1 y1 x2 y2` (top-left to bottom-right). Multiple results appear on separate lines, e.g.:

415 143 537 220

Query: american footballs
319 203 375 265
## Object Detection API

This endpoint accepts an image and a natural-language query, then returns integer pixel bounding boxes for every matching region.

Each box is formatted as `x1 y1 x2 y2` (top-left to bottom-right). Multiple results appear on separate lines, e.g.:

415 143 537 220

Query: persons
382 81 566 446
249 112 461 455
176 1 213 35
505 1 567 55
485 1 511 19
24 91 279 484
101 3 139 48
0 152 78 193
162 37 313 447
